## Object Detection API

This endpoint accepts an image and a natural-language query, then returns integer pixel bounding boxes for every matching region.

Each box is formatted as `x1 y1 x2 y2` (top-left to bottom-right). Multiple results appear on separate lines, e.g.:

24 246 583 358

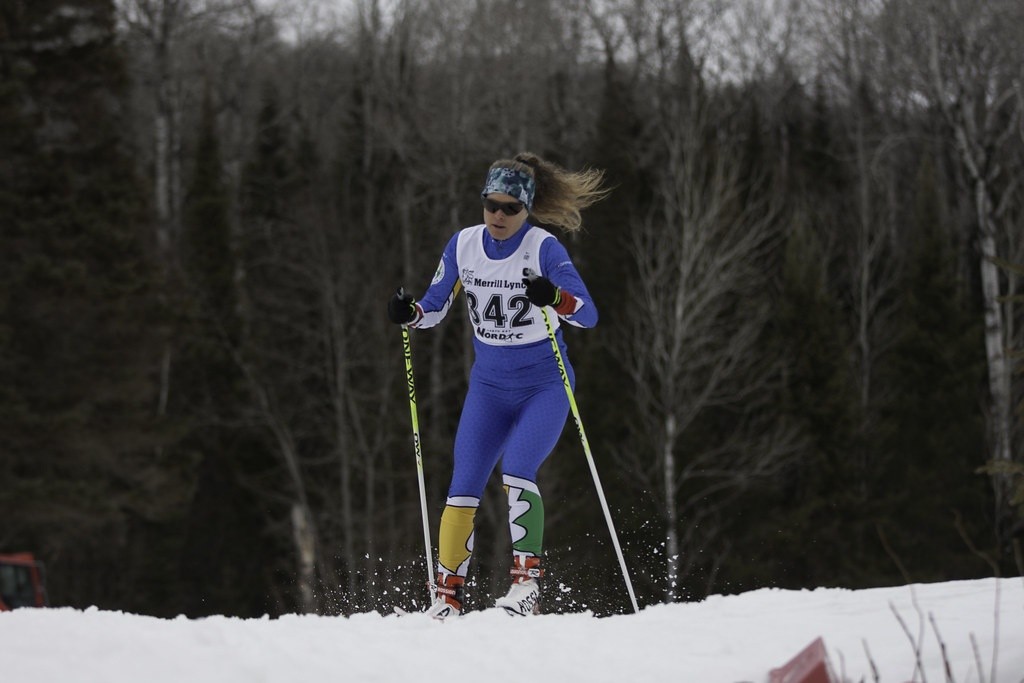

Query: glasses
481 197 526 216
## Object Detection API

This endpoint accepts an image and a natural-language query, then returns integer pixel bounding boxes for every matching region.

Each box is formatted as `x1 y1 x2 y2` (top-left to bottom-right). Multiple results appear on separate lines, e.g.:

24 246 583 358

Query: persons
388 152 608 615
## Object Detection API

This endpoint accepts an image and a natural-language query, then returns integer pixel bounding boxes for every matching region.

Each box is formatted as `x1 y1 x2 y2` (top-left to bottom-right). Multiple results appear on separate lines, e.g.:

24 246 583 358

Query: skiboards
391 604 539 623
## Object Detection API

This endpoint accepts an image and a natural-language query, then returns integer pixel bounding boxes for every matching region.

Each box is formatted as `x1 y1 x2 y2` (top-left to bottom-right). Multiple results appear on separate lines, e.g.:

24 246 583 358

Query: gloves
387 292 417 324
522 268 561 308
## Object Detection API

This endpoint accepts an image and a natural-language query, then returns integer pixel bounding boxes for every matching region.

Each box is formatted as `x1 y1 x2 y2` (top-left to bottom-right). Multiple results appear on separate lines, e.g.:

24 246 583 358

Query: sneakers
495 578 541 616
425 597 462 621
